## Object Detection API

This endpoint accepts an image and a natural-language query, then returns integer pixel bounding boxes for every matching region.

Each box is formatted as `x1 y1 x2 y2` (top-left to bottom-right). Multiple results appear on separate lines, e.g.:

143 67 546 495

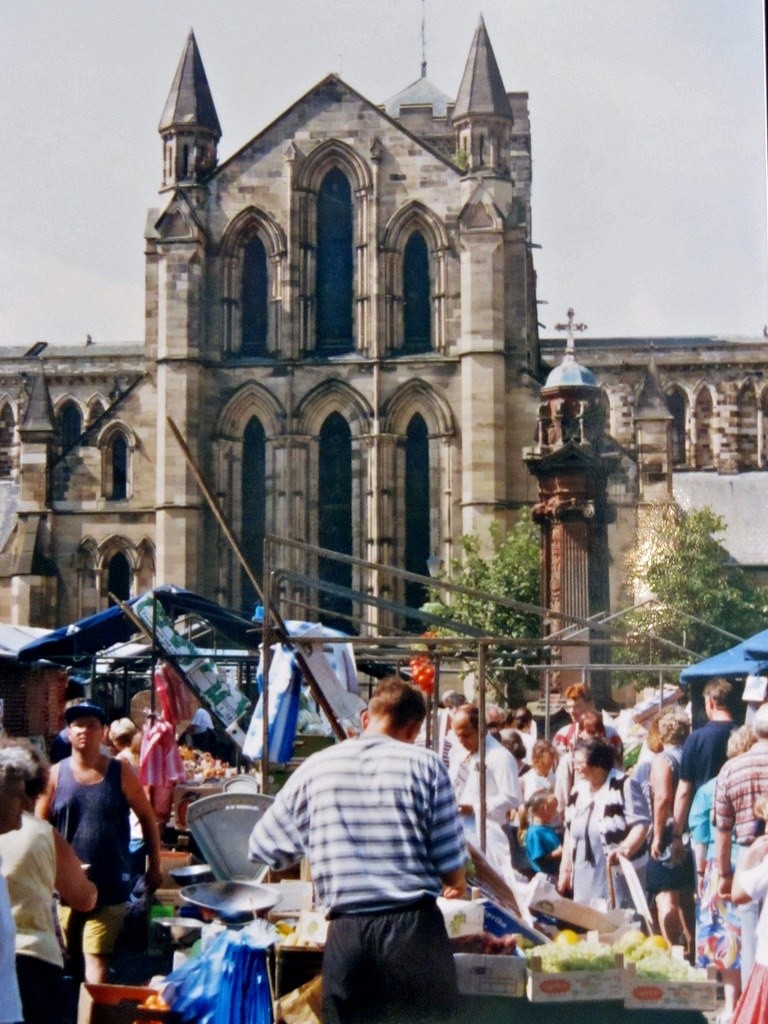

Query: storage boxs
156 850 193 905
451 890 717 1012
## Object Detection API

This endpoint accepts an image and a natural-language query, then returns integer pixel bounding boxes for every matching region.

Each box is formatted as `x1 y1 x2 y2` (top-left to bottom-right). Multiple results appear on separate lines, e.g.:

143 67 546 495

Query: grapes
529 941 707 981
450 933 517 956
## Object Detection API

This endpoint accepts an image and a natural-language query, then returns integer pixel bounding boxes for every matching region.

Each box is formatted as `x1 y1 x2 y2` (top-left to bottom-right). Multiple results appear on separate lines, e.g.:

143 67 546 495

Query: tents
680 625 768 731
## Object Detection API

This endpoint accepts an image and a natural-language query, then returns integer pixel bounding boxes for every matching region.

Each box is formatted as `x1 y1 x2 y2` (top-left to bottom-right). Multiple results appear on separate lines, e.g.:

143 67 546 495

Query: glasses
572 758 587 766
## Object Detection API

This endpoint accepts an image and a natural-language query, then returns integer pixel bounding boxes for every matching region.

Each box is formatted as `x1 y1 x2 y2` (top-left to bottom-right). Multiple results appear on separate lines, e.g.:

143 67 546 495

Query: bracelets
719 872 732 877
697 872 703 874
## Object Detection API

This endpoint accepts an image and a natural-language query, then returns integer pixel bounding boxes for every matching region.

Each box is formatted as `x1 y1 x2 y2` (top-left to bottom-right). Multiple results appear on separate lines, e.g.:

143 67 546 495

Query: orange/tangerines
408 657 435 694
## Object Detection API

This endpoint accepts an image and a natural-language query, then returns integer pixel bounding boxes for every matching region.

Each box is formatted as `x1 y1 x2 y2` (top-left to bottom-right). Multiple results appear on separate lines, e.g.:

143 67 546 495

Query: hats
63 701 105 725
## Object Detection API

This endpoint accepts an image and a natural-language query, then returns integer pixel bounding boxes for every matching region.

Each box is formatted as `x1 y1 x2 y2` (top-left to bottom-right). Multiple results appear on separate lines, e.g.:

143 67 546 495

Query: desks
268 910 325 1024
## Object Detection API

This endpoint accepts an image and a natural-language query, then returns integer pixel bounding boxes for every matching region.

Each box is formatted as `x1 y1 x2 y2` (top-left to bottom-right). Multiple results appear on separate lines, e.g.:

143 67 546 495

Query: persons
427 689 517 866
484 705 537 775
245 674 469 1024
633 679 768 1024
554 684 624 805
177 708 220 757
517 742 557 801
557 739 648 918
53 699 146 872
524 788 563 879
33 702 160 1024
0 746 97 1024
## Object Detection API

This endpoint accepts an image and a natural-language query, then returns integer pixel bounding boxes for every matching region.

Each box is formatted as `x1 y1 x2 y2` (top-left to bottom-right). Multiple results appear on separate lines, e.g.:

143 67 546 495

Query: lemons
557 929 580 945
622 930 668 952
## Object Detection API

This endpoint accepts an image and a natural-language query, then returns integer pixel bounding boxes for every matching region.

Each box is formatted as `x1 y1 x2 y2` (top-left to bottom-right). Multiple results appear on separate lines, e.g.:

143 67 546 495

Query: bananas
275 920 321 947
